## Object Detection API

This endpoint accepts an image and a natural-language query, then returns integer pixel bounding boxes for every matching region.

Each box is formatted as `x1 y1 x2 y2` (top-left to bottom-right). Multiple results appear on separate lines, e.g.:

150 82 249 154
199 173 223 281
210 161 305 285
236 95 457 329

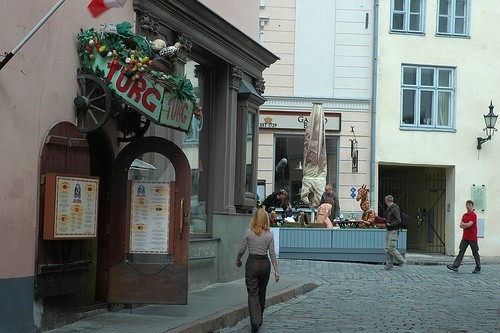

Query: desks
333 220 364 228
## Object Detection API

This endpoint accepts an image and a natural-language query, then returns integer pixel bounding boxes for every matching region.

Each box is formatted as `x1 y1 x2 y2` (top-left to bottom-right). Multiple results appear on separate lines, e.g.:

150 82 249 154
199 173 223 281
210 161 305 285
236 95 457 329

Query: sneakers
447 265 458 271
472 270 479 273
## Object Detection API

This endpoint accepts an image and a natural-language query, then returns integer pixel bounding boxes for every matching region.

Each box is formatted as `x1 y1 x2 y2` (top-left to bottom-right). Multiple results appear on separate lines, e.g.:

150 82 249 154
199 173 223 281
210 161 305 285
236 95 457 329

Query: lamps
294 161 303 170
477 99 498 149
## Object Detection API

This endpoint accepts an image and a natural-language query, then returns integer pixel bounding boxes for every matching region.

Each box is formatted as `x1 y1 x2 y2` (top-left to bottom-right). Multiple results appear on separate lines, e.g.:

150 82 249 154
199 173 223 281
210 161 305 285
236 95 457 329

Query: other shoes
399 261 406 269
380 266 393 270
251 324 258 333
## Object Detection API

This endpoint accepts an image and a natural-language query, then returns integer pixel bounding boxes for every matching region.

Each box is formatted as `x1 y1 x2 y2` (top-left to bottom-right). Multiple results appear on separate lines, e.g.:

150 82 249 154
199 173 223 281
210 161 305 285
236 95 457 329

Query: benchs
284 212 327 228
